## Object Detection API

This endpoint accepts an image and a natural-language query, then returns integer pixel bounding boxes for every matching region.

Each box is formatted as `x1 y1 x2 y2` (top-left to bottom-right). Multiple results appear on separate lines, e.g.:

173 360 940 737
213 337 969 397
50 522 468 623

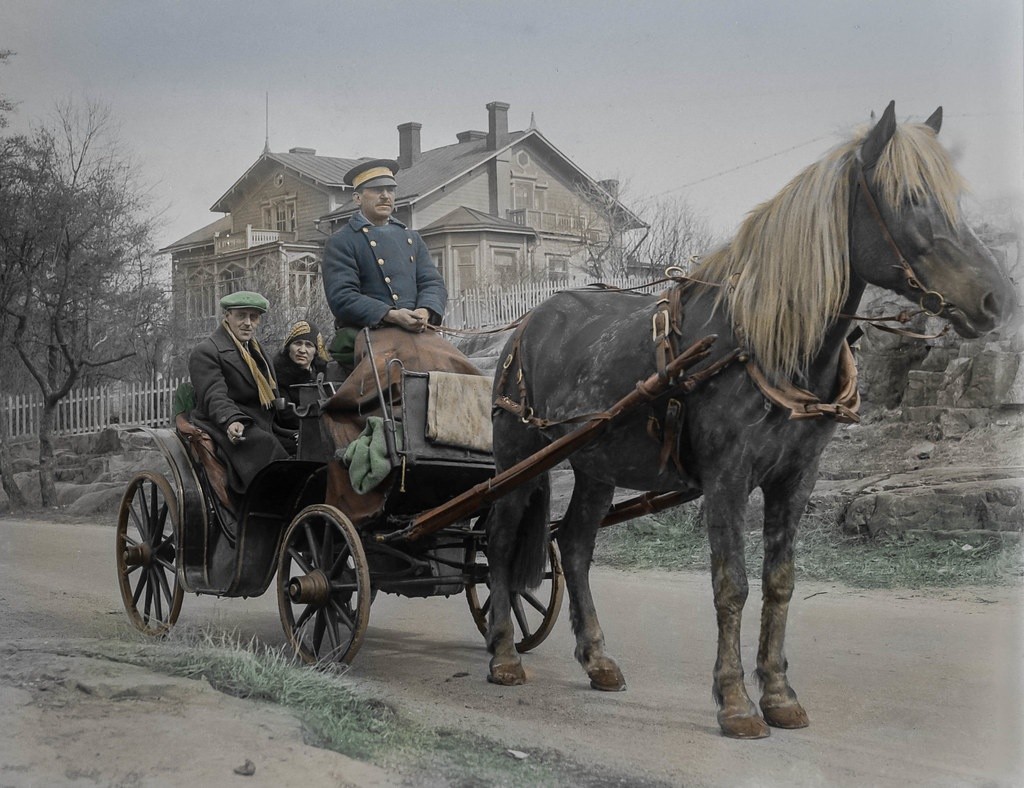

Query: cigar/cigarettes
232 436 236 441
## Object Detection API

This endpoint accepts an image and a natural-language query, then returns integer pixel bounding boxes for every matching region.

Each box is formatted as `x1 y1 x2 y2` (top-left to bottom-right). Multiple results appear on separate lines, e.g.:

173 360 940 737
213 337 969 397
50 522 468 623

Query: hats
220 290 271 313
343 159 399 191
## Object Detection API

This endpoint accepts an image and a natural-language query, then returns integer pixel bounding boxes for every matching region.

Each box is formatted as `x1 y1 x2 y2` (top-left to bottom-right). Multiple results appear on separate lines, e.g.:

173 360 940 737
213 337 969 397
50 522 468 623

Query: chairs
172 385 266 519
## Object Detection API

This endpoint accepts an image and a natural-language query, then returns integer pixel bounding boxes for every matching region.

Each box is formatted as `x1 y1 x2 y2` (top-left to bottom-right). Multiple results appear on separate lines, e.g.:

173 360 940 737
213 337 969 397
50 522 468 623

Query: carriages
115 96 1012 738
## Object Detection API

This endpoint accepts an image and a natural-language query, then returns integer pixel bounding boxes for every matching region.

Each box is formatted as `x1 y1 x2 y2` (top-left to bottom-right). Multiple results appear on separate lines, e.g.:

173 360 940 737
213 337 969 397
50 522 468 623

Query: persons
188 290 301 518
319 159 449 369
272 319 330 428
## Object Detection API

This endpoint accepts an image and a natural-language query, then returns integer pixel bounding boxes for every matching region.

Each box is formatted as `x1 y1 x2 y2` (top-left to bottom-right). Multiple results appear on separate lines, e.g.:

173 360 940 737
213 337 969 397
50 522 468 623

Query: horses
484 98 1017 742
